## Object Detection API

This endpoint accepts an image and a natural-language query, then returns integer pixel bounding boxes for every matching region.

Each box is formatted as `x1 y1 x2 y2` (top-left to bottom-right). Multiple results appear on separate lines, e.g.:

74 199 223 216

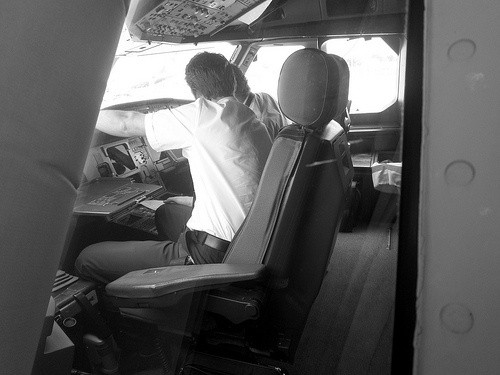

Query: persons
75 50 276 355
228 63 291 148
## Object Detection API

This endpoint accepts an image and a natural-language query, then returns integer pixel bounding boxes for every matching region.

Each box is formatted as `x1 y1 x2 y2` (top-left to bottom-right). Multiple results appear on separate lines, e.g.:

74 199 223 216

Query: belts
195 230 230 253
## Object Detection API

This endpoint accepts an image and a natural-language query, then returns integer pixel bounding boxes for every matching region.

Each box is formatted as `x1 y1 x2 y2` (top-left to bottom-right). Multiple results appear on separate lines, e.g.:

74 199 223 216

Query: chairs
105 47 354 375
